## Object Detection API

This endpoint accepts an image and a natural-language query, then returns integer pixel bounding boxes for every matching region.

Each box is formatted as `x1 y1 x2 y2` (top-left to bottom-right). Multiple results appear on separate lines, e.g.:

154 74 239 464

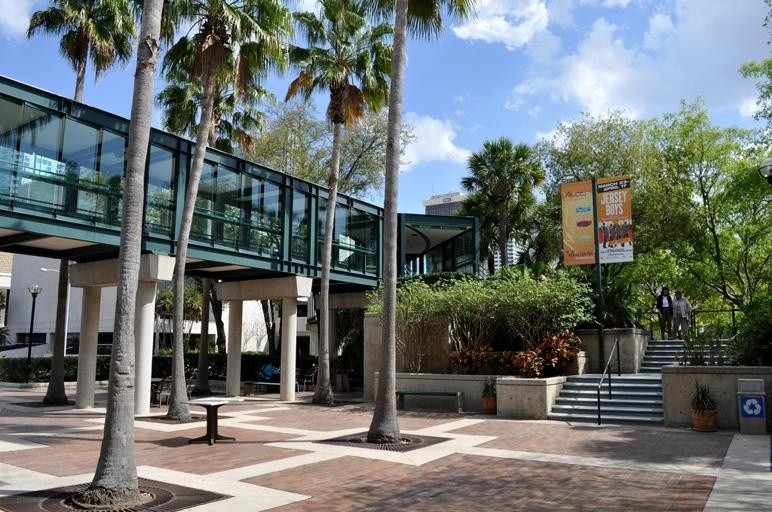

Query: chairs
151 377 194 408
254 367 318 393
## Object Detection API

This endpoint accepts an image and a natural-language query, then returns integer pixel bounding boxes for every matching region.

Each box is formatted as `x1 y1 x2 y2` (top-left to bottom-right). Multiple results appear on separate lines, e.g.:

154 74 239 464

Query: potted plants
481 380 496 414
689 379 719 432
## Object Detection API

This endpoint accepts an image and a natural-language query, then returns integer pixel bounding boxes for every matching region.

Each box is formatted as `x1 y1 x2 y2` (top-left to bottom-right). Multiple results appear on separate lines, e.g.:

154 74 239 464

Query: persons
602 223 609 249
622 221 631 247
614 224 624 247
656 286 673 340
609 223 616 248
670 290 693 341
259 361 280 394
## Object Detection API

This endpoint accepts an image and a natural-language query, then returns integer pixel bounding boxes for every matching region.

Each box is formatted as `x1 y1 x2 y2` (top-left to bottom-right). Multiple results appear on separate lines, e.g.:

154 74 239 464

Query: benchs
244 381 280 397
396 391 465 414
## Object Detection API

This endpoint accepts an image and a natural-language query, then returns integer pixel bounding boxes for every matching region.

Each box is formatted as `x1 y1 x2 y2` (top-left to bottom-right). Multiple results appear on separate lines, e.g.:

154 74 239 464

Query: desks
179 396 245 445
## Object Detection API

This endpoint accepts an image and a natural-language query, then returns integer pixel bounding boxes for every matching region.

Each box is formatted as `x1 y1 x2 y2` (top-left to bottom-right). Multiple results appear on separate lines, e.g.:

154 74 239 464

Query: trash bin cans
244 381 256 397
735 379 767 435
335 368 353 392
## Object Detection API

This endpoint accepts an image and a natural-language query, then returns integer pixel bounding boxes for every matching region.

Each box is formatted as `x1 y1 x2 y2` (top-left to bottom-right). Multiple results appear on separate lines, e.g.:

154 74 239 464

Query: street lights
41 268 70 355
25 284 43 382
